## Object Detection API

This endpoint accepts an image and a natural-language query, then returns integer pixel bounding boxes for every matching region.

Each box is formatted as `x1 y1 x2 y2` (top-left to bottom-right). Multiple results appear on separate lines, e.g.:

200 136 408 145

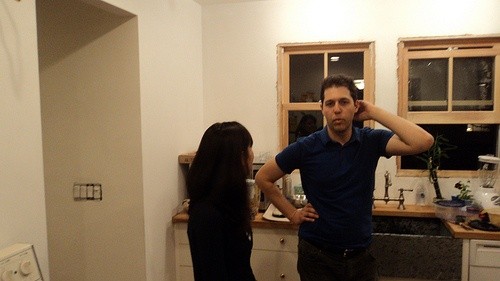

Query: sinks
364 214 453 235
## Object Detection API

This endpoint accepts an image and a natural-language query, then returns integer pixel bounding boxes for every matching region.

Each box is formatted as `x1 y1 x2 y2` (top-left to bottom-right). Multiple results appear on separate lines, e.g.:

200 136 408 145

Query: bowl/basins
433 201 466 220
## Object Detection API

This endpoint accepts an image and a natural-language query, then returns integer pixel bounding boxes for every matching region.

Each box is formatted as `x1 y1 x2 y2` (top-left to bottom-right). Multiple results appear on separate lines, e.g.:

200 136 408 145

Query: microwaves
253 161 276 212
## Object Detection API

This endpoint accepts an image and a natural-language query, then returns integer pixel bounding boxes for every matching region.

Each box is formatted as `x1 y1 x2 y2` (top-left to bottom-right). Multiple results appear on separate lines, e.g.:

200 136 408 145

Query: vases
452 196 473 206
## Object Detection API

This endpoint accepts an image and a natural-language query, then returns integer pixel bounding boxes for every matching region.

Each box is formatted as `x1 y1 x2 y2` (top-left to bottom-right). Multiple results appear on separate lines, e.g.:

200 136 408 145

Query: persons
255 75 435 281
296 114 317 140
187 122 256 281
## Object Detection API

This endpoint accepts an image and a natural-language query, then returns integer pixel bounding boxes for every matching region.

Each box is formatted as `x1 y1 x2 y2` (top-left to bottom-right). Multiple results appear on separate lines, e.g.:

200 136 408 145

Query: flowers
454 180 474 200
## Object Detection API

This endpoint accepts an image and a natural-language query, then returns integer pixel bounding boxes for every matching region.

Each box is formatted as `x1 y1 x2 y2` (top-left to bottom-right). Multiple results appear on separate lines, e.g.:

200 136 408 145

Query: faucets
384 171 392 200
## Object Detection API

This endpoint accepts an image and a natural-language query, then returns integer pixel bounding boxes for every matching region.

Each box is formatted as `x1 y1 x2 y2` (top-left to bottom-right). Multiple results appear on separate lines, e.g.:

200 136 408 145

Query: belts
303 237 368 260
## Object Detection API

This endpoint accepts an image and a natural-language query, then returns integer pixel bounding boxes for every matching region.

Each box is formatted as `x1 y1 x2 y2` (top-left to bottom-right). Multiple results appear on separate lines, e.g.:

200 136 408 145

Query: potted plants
414 136 446 202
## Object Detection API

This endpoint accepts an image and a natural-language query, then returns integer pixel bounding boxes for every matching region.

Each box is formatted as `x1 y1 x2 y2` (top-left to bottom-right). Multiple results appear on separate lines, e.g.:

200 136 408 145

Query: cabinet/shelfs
462 239 500 281
174 223 300 281
